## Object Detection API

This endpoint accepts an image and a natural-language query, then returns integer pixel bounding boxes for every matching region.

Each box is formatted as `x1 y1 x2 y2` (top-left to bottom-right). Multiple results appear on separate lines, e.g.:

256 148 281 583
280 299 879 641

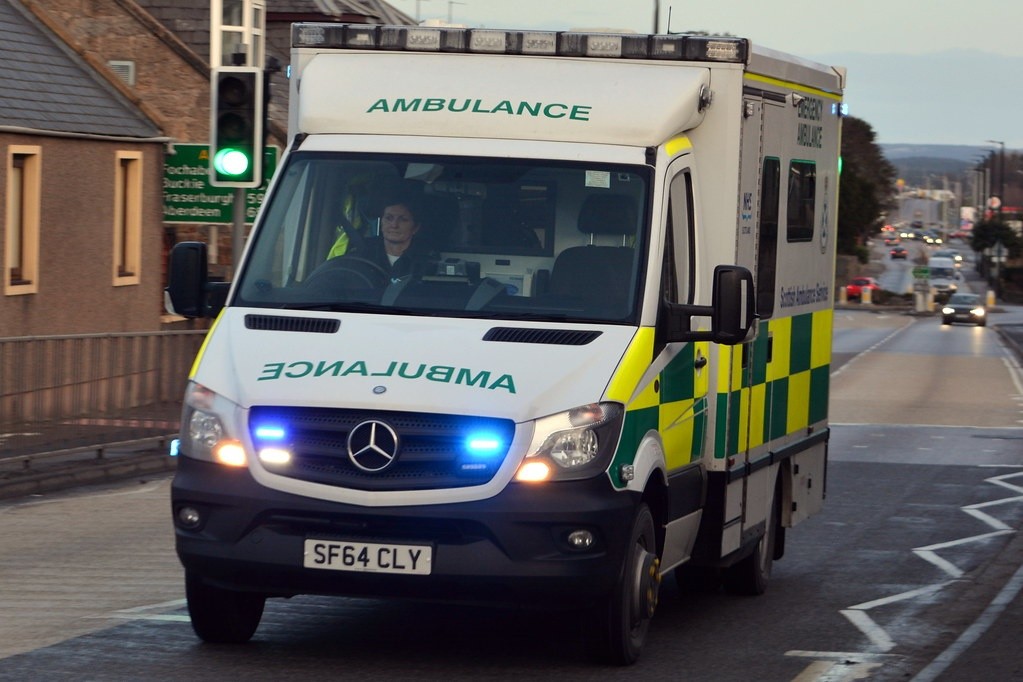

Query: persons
336 193 442 308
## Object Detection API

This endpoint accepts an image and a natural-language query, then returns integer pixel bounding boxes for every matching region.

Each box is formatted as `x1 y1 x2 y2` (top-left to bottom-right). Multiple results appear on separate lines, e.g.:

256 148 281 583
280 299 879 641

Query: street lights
969 139 1005 281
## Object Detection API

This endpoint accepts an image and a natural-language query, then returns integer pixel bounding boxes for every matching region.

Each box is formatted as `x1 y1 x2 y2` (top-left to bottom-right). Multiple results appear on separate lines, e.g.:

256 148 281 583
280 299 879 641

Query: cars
942 293 986 325
891 247 907 260
934 250 962 261
848 278 880 302
881 224 942 245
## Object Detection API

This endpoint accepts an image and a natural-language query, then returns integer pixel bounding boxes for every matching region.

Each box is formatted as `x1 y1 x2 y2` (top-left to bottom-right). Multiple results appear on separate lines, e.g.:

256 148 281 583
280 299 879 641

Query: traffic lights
211 67 261 190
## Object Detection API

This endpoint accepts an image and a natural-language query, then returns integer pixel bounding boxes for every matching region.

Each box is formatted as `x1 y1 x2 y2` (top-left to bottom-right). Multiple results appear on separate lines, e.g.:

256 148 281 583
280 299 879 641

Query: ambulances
171 22 845 665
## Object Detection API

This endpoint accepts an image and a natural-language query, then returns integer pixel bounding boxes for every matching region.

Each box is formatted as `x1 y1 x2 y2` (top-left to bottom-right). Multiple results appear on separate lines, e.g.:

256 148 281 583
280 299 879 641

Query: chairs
550 192 637 319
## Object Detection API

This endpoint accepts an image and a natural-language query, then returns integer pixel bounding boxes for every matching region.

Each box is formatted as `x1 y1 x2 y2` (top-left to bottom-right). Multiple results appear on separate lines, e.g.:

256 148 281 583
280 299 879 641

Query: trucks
929 257 957 292
